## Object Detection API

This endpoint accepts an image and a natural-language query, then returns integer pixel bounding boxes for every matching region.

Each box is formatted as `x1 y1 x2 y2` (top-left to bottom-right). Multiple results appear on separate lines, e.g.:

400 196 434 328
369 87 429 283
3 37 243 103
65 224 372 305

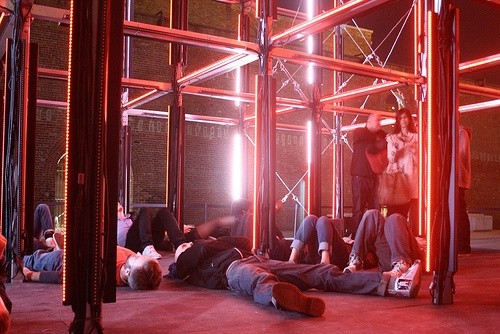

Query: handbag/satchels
378 160 411 204
366 148 389 174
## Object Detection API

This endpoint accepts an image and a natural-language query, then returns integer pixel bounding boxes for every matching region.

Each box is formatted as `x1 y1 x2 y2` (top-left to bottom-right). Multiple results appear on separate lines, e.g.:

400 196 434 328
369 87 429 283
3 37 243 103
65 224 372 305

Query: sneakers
343 256 364 273
272 282 326 317
142 245 162 259
383 261 410 277
388 259 422 298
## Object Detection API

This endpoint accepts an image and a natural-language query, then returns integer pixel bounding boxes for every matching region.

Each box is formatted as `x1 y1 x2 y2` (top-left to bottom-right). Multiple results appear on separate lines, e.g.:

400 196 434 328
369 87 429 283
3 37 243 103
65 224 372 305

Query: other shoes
458 249 470 254
53 233 64 250
343 235 352 243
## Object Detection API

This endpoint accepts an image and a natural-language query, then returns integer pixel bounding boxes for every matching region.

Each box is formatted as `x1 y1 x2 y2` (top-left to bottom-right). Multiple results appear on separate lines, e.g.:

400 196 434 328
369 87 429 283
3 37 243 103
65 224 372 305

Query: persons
20 244 161 290
185 197 291 260
457 111 471 257
34 203 64 250
118 201 188 258
169 236 422 316
350 113 387 239
343 209 426 277
385 108 418 236
289 215 377 270
